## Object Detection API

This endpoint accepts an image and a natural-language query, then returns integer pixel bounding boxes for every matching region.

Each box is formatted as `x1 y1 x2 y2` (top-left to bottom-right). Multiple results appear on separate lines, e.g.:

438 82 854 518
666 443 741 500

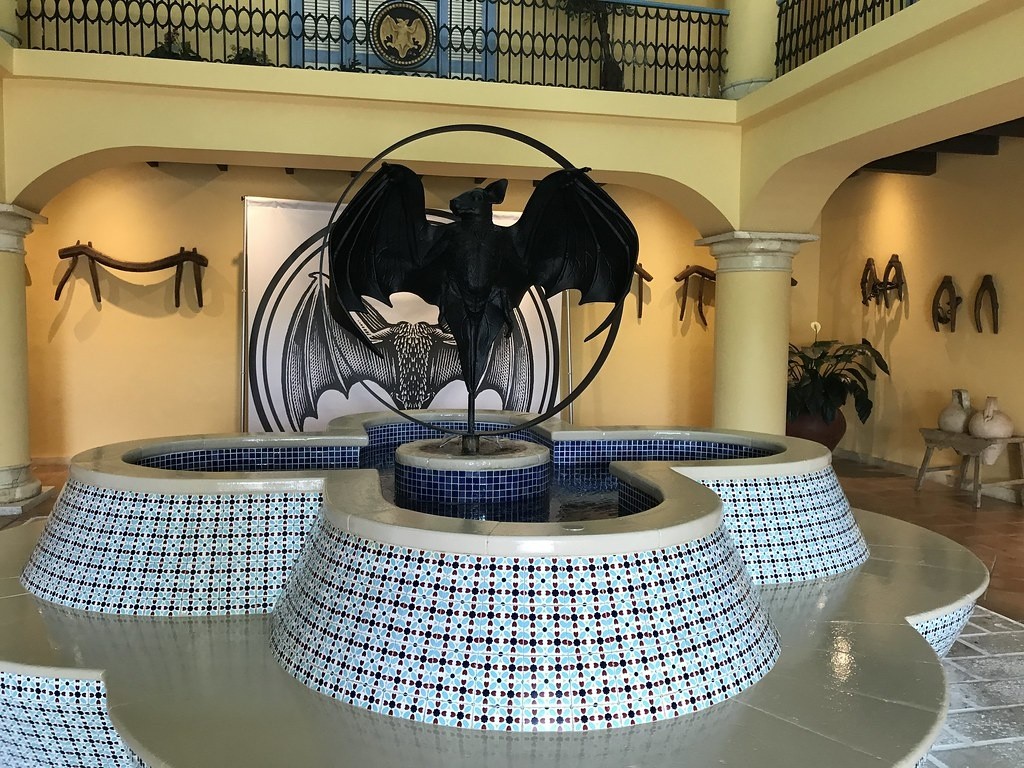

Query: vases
967 396 1014 438
787 405 848 457
936 389 976 433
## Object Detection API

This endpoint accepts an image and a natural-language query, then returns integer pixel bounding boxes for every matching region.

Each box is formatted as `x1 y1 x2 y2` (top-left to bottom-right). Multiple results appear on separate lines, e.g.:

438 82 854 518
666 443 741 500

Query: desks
914 426 1023 509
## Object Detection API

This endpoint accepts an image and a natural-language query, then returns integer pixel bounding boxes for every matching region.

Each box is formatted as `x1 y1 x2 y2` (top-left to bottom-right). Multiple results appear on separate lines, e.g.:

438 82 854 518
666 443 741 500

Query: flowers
786 320 893 425
